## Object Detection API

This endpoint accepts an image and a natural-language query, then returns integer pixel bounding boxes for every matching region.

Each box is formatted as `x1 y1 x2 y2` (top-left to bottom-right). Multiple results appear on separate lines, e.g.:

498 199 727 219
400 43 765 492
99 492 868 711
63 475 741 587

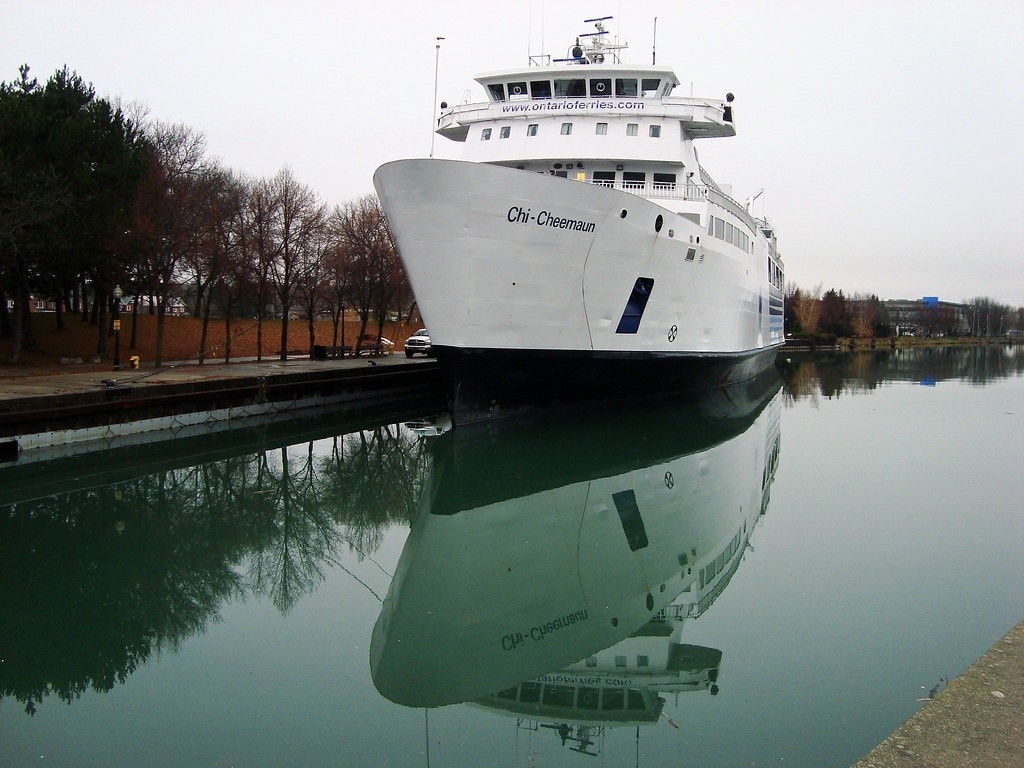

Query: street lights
112 284 122 371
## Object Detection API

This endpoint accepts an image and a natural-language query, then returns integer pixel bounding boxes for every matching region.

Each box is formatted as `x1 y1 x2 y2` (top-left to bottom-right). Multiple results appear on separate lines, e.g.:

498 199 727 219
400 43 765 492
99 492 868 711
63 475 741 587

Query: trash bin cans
388 344 395 355
130 355 140 369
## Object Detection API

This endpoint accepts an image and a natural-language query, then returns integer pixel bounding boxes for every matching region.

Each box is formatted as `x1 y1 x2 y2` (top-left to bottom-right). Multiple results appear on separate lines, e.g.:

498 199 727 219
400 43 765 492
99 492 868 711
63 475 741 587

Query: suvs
404 329 435 359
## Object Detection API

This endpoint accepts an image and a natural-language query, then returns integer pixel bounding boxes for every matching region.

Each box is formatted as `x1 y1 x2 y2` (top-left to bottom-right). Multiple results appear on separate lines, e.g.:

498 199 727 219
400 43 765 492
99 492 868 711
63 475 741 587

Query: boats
372 16 787 411
368 366 786 768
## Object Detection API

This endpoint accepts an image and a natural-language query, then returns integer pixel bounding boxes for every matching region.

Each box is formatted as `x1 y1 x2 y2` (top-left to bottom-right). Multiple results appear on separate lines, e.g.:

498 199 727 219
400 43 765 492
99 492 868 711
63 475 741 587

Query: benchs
326 342 388 360
